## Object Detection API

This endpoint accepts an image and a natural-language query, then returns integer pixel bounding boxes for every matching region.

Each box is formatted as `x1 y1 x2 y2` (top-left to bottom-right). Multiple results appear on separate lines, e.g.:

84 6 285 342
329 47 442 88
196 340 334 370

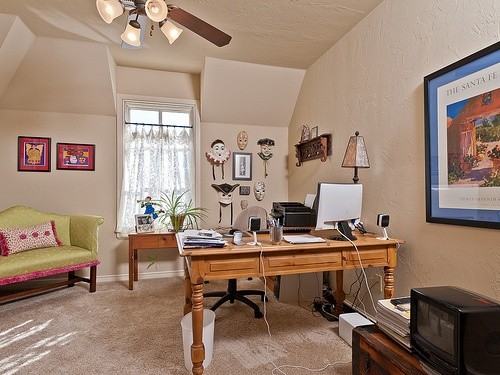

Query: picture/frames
17 136 52 172
232 151 253 180
56 143 96 171
424 42 500 230
135 213 153 233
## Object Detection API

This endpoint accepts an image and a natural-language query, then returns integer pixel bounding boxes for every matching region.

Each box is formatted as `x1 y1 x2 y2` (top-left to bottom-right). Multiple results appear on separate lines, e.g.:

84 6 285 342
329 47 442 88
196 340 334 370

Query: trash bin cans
180 309 215 371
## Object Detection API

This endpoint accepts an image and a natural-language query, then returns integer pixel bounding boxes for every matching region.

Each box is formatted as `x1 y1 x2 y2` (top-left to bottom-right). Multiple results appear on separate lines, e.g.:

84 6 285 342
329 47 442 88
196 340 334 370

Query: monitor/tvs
410 285 500 375
315 182 363 241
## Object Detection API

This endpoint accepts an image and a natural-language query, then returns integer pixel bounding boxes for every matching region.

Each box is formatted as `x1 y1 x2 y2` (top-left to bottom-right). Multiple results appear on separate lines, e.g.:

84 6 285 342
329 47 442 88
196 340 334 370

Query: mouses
229 229 242 235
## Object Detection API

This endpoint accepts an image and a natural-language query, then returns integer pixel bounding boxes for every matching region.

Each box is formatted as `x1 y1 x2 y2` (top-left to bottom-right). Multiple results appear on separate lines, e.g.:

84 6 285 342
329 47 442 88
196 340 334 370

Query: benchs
0 205 104 305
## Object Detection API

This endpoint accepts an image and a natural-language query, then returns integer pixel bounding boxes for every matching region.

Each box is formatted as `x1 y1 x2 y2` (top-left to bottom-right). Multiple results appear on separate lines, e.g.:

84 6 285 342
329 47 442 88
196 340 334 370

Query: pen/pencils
267 218 280 227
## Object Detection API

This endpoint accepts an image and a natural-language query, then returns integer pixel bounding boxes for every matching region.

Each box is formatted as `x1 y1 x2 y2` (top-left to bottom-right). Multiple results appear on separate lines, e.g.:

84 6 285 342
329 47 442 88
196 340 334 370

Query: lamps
94 0 183 47
341 132 370 184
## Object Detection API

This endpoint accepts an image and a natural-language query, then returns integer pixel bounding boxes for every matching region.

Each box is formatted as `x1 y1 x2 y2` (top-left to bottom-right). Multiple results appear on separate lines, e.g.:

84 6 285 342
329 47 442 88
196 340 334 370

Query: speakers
247 215 261 245
376 214 390 240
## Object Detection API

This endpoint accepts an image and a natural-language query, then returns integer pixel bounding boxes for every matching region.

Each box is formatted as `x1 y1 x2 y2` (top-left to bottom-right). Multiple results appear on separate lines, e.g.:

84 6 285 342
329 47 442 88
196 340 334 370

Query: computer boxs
274 271 323 302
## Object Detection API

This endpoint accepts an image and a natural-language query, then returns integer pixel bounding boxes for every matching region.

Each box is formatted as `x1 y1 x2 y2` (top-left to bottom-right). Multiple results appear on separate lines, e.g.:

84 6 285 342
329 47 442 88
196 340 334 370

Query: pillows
0 221 62 257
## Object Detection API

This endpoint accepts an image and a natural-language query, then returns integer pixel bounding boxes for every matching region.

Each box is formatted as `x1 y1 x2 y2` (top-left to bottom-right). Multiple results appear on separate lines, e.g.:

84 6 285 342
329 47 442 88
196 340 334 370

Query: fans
121 0 232 50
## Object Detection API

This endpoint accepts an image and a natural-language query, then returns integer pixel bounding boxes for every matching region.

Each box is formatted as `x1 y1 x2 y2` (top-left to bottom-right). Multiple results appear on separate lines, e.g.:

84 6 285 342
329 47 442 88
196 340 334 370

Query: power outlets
375 274 381 292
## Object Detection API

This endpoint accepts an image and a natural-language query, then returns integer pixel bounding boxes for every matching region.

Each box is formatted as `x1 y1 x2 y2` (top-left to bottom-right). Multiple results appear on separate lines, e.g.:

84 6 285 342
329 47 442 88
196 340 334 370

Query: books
375 296 410 339
182 230 225 249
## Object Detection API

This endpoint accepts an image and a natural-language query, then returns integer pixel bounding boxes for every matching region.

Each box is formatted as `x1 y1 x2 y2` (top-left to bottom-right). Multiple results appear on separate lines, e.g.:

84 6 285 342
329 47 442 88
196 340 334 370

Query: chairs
202 205 271 318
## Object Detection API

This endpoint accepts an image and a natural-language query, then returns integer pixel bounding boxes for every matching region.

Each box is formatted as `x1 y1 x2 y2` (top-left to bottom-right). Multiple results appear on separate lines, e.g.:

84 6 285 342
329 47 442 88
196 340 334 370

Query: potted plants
141 187 207 229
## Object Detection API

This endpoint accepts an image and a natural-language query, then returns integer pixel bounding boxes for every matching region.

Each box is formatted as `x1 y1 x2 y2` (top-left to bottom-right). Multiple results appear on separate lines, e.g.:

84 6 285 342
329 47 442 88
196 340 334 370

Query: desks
174 228 405 374
352 324 437 375
128 229 190 290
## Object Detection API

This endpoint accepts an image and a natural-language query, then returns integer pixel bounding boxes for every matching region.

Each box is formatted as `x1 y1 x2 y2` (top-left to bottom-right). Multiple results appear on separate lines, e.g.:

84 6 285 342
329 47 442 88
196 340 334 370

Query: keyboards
247 229 269 234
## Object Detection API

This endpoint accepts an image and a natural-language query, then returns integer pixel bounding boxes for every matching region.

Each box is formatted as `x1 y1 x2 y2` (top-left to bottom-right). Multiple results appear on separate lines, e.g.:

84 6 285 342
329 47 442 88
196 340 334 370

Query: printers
270 198 317 228
